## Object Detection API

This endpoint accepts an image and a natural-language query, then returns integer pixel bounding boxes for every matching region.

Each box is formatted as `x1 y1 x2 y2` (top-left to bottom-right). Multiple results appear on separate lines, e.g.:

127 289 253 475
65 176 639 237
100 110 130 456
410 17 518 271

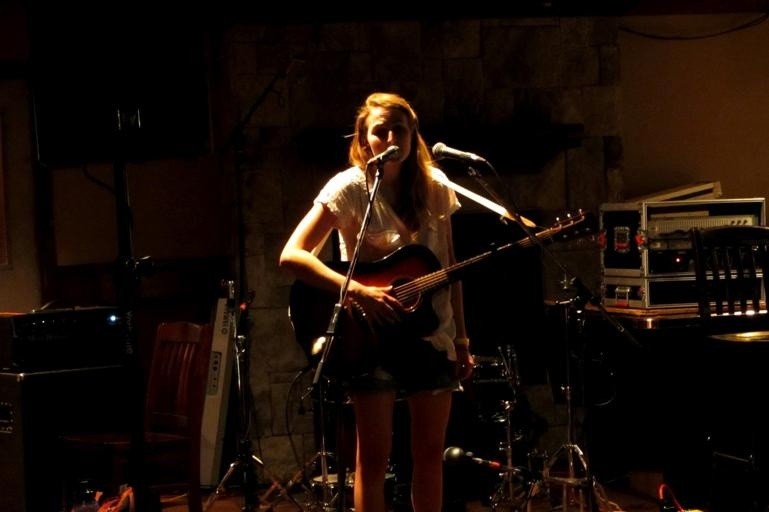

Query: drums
308 471 398 511
462 355 509 385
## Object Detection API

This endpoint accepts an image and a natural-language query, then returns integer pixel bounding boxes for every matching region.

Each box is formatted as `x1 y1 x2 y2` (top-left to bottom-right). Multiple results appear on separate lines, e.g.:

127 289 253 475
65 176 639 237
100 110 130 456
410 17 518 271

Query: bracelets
455 336 469 345
456 348 470 352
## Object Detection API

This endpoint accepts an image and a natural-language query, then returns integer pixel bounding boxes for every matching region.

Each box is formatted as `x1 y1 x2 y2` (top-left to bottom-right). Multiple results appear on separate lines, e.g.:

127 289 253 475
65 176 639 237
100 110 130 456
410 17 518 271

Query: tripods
196 337 298 512
519 361 620 512
262 385 349 512
484 389 535 512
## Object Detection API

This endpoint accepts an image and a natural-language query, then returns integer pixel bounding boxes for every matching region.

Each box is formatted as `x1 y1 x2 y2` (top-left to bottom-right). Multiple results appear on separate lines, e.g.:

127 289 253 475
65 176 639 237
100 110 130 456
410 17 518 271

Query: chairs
58 318 209 512
690 224 768 511
285 258 360 493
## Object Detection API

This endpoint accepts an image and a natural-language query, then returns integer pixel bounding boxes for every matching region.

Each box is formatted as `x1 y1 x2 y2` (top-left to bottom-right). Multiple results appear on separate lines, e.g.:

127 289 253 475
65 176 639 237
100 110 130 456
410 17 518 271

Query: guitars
290 209 601 378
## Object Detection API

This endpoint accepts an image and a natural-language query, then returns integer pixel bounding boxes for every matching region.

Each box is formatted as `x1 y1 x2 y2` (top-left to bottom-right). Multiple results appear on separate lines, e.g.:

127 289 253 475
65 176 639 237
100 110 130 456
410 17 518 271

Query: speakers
0 366 163 512
710 451 769 511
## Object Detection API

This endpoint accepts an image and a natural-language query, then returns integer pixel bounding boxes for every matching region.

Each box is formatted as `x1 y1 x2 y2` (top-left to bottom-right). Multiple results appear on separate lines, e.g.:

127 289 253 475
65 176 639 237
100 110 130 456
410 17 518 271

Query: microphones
429 140 489 170
438 441 511 471
365 143 405 166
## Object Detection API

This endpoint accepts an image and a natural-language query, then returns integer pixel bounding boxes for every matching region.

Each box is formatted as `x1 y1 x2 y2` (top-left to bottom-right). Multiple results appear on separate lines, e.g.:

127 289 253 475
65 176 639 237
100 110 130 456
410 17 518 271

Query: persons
279 92 476 511
